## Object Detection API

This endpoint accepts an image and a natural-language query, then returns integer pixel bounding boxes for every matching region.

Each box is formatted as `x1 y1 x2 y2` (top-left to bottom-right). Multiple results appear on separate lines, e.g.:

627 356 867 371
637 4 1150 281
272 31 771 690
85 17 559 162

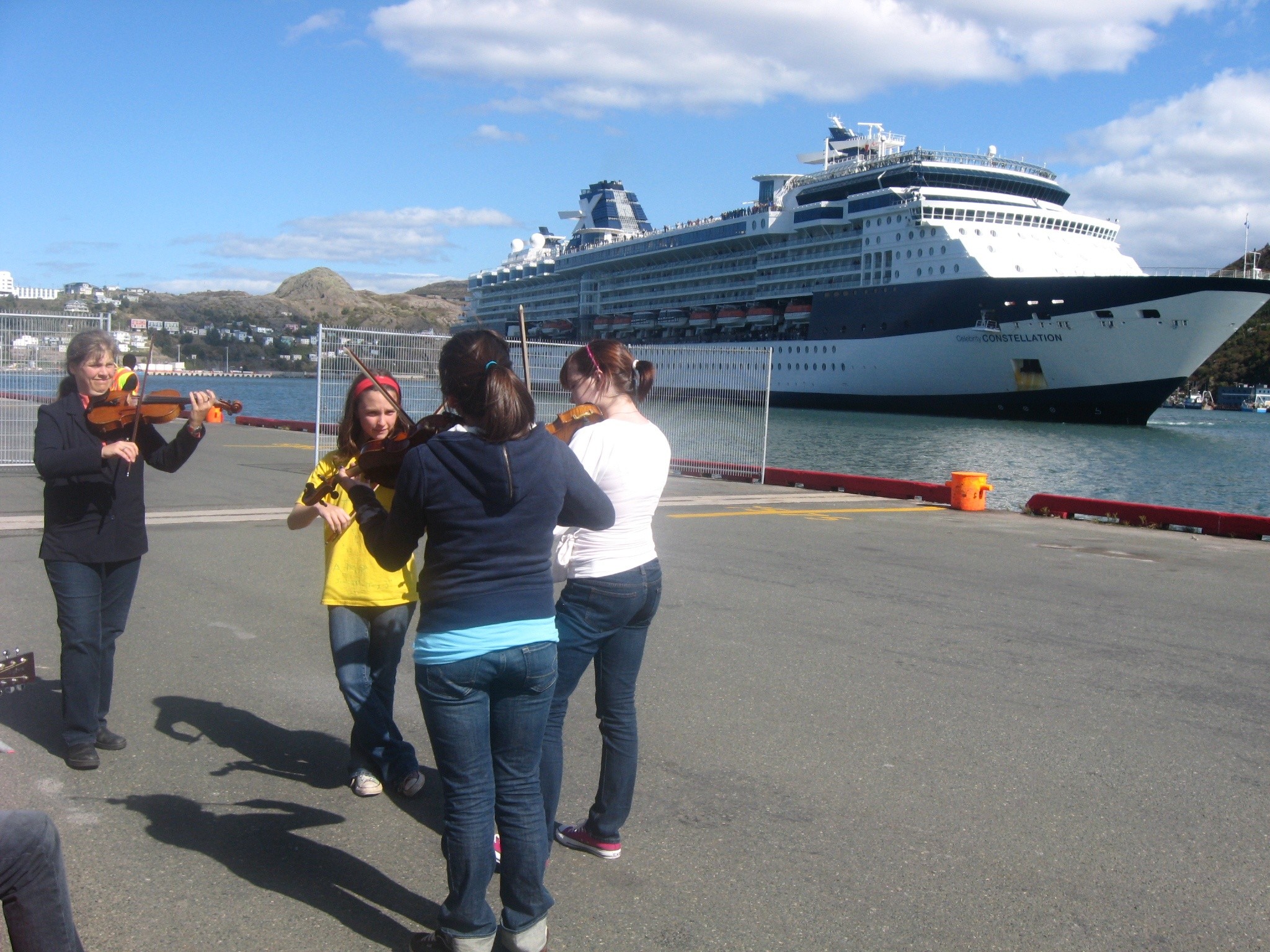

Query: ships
446 111 1270 428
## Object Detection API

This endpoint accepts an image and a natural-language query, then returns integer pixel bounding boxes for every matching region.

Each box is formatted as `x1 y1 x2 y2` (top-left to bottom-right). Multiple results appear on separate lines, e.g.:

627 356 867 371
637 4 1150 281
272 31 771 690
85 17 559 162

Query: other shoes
95 727 126 750
66 742 100 767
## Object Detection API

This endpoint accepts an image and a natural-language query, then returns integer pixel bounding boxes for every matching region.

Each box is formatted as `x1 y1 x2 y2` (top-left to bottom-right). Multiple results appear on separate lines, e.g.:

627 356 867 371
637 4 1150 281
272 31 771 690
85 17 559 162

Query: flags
1245 217 1250 229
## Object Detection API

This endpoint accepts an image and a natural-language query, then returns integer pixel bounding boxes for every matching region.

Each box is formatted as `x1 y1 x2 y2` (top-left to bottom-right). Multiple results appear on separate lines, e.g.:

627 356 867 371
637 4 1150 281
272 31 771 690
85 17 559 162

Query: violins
544 401 604 446
86 389 242 433
302 411 466 507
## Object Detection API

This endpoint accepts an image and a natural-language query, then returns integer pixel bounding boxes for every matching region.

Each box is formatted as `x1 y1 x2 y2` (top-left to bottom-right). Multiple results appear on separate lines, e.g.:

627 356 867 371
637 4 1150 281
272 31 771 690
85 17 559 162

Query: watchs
187 425 202 435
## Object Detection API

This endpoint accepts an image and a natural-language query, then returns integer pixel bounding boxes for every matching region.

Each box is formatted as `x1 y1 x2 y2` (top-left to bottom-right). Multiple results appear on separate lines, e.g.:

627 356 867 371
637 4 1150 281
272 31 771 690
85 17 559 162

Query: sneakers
493 833 502 863
555 818 622 860
409 927 452 952
350 775 383 796
397 770 425 797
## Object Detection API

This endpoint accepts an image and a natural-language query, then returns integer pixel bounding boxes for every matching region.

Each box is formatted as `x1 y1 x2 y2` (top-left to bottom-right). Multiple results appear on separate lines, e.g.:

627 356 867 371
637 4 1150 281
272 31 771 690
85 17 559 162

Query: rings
122 447 127 452
204 397 209 403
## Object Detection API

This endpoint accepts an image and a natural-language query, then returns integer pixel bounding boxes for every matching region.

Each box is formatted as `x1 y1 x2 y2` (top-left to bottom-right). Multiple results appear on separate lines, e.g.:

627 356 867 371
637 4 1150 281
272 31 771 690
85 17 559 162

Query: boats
539 297 811 341
1240 395 1267 413
1158 380 1203 409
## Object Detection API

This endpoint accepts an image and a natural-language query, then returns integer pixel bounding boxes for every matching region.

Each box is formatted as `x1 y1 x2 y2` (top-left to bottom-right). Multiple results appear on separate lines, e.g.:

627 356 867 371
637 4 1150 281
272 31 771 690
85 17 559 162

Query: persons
516 254 518 261
288 370 427 800
843 225 861 236
1106 218 1119 224
332 326 614 952
463 255 836 347
493 338 672 864
33 330 216 768
910 192 924 202
788 151 935 188
545 200 782 257
109 354 139 395
0 811 84 952
